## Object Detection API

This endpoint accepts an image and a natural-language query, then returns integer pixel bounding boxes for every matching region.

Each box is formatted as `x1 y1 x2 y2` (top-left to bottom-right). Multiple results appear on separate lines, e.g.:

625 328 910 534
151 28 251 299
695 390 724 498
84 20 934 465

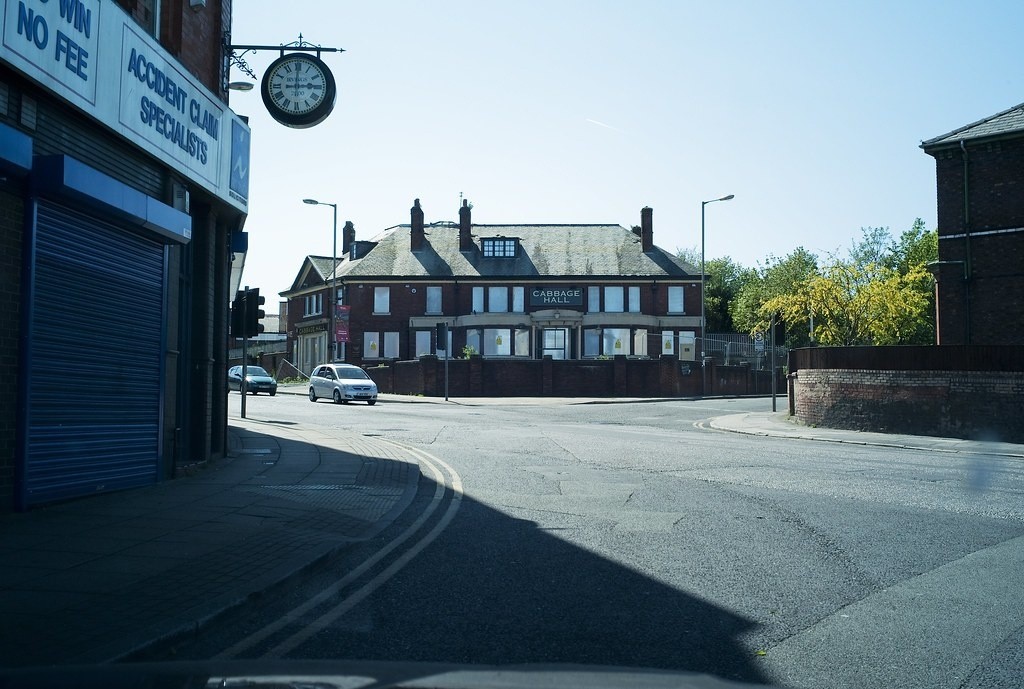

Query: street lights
302 199 337 363
701 193 734 397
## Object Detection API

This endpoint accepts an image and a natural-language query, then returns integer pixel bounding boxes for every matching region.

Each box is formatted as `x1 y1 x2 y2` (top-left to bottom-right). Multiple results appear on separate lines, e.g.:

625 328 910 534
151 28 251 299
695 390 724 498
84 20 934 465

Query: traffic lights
249 287 265 337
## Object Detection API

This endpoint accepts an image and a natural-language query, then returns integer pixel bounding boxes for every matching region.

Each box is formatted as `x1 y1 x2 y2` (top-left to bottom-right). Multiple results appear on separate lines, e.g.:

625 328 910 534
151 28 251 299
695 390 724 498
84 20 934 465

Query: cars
309 363 378 406
228 365 278 396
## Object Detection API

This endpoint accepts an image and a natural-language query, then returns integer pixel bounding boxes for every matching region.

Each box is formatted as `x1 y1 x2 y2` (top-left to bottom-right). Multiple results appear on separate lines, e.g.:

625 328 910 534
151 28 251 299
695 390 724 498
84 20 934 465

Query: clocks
260 53 338 130
